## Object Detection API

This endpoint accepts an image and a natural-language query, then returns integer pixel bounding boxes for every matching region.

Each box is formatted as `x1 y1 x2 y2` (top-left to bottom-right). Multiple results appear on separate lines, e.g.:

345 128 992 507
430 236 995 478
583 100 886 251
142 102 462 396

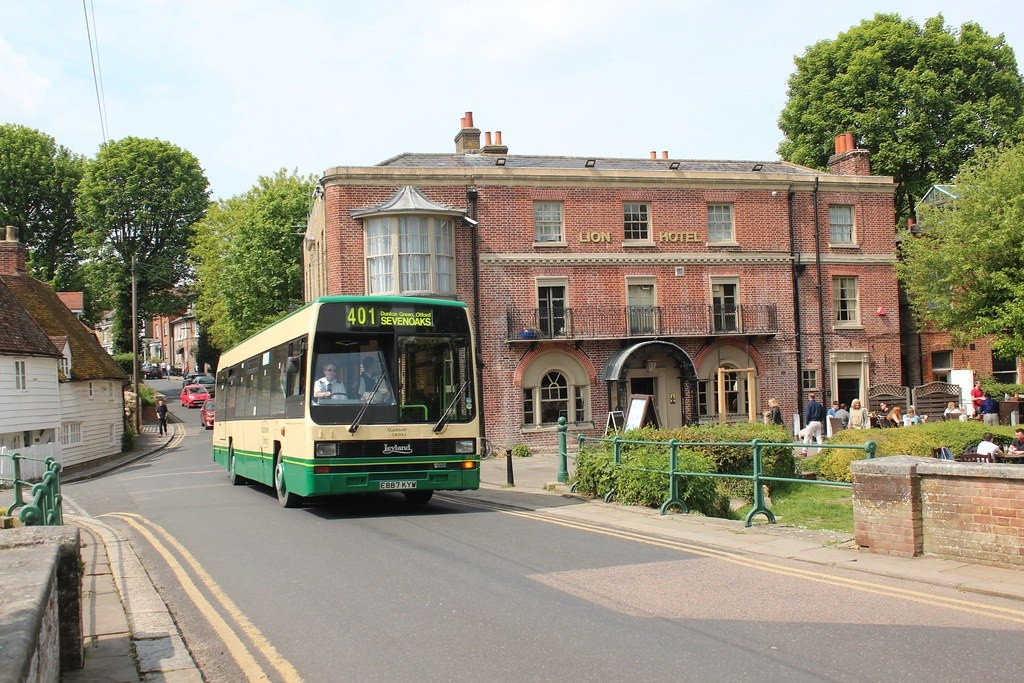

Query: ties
327 383 332 393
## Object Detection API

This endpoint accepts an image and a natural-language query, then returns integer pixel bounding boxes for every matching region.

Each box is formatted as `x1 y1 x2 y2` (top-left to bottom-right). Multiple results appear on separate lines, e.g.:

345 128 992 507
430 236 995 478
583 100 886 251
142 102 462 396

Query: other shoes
164 431 168 436
157 433 162 437
799 452 807 457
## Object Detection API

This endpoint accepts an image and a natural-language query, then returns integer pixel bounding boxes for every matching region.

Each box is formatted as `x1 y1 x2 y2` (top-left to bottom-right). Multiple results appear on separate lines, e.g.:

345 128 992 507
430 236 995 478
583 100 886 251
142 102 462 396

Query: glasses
326 369 337 373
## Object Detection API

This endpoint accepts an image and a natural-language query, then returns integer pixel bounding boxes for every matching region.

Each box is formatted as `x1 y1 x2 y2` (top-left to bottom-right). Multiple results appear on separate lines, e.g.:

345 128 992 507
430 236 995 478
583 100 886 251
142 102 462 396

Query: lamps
585 160 595 168
496 158 506 166
623 365 629 374
752 164 763 171
644 360 659 373
669 163 680 169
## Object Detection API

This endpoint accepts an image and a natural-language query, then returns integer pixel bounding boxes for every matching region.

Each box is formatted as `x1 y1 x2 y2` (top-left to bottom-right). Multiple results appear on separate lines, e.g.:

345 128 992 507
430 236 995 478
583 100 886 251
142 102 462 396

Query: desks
996 450 1024 464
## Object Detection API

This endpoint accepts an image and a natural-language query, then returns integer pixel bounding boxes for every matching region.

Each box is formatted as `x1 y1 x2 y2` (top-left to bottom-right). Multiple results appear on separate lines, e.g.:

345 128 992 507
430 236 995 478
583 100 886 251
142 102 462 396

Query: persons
943 401 960 418
765 397 784 427
797 392 926 457
141 360 167 379
357 357 378 401
976 432 1003 463
977 393 1000 427
313 361 347 404
1010 428 1024 464
971 380 986 414
156 400 168 437
174 361 189 379
166 364 171 380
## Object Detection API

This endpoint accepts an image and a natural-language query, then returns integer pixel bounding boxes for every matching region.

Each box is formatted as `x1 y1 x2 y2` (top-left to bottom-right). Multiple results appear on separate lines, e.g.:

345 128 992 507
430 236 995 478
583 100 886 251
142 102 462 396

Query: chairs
793 413 991 462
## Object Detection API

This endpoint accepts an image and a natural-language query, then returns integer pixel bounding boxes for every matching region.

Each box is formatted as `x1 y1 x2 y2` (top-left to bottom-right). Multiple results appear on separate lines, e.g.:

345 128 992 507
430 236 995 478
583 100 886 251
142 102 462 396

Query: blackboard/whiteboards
622 395 661 435
604 411 625 436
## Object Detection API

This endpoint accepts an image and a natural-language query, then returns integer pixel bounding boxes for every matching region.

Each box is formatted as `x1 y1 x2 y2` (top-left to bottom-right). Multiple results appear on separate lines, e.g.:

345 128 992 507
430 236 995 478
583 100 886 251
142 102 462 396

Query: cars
180 385 211 406
140 366 164 380
200 399 215 429
183 374 207 389
192 376 216 395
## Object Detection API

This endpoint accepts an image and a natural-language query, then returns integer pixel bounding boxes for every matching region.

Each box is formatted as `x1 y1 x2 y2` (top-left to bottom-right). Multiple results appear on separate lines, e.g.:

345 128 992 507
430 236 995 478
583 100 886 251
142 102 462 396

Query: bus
212 294 485 509
